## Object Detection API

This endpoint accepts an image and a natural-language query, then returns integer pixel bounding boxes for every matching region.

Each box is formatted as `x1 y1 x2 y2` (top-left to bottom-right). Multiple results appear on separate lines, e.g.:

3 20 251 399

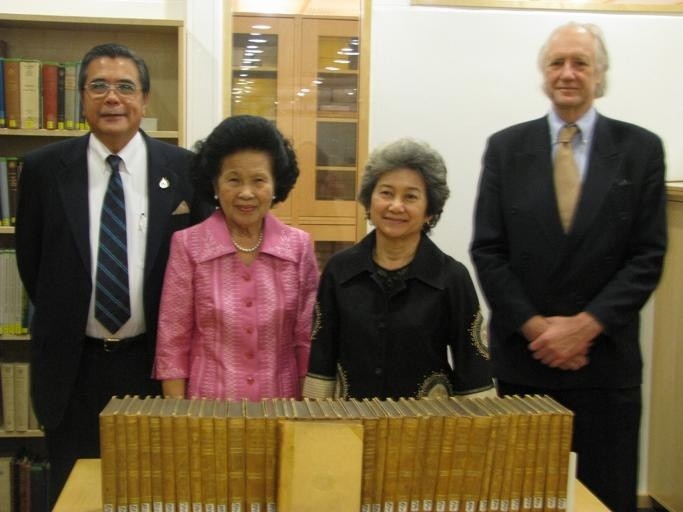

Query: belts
83 334 147 351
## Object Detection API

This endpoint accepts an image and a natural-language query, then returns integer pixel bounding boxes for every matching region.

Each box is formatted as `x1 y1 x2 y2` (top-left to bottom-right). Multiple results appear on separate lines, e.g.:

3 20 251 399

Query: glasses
81 82 142 94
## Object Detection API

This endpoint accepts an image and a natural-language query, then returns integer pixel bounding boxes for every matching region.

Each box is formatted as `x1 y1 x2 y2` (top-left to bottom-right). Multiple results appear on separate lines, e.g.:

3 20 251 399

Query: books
316 111 356 119
141 118 158 131
0 250 30 336
1 364 38 434
0 452 53 511
1 160 23 227
1 56 92 131
100 396 575 512
318 105 350 112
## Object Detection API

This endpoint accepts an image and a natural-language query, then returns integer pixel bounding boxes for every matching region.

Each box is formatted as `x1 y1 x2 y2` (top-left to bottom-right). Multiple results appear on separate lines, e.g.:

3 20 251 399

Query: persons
304 136 495 400
150 113 319 401
466 20 667 512
14 42 217 491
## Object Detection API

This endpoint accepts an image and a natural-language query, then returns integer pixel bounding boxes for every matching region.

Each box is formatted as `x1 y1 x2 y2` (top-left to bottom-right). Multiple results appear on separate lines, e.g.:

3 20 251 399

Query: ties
94 155 131 335
552 126 582 234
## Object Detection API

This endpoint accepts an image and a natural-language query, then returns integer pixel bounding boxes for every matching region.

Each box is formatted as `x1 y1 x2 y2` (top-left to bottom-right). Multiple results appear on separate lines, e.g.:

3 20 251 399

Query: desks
51 459 611 512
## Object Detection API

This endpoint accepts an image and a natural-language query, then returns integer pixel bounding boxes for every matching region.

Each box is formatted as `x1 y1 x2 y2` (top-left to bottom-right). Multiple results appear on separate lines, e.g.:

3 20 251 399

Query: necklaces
232 233 264 252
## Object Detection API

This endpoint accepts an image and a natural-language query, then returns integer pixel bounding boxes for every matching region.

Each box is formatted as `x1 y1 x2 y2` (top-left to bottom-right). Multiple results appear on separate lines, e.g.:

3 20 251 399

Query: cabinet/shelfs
1 13 186 512
222 0 367 275
